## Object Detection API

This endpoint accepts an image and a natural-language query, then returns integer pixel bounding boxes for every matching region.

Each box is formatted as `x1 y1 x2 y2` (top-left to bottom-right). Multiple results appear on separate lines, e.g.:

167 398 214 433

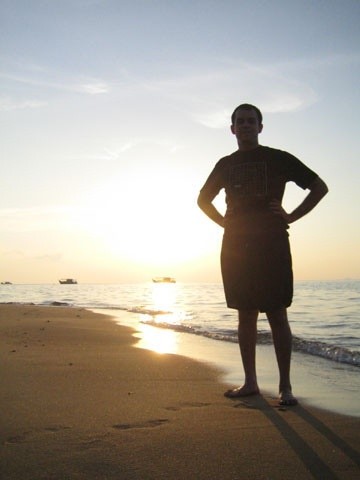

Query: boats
152 278 176 283
1 282 12 284
59 279 77 284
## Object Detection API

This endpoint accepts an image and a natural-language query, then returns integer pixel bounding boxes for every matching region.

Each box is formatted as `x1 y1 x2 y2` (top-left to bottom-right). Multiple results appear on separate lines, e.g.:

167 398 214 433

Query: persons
196 104 329 406
